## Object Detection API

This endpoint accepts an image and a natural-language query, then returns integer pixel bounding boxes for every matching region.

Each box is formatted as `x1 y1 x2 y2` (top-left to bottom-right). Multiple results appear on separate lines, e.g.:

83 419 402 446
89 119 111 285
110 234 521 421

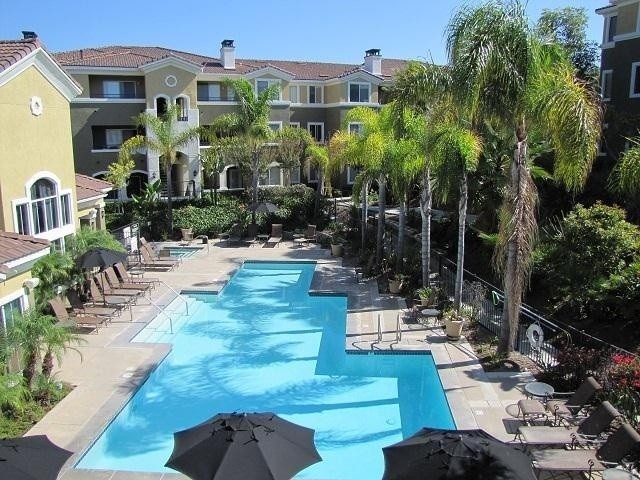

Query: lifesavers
527 324 543 347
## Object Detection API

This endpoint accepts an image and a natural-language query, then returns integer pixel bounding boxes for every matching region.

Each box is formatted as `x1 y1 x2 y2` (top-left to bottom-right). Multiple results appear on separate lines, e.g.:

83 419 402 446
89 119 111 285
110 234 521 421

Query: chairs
267 224 282 248
48 236 182 333
307 224 317 245
228 224 241 247
513 377 640 479
243 224 256 247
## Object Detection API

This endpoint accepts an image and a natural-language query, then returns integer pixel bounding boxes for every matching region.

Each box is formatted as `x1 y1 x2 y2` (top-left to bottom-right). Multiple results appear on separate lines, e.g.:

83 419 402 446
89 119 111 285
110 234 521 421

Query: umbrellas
0 435 76 480
378 424 535 480
74 246 129 303
243 200 280 215
163 410 325 480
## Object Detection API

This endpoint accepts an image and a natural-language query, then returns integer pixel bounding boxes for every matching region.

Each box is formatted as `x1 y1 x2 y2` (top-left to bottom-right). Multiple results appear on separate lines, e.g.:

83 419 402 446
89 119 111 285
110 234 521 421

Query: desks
293 234 306 245
422 308 441 329
294 238 307 255
257 233 269 244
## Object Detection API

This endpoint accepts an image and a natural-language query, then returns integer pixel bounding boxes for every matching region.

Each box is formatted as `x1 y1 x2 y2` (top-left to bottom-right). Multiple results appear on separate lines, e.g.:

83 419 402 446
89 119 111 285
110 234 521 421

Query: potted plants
388 272 404 294
416 286 431 306
444 310 464 341
171 206 200 241
330 234 343 256
